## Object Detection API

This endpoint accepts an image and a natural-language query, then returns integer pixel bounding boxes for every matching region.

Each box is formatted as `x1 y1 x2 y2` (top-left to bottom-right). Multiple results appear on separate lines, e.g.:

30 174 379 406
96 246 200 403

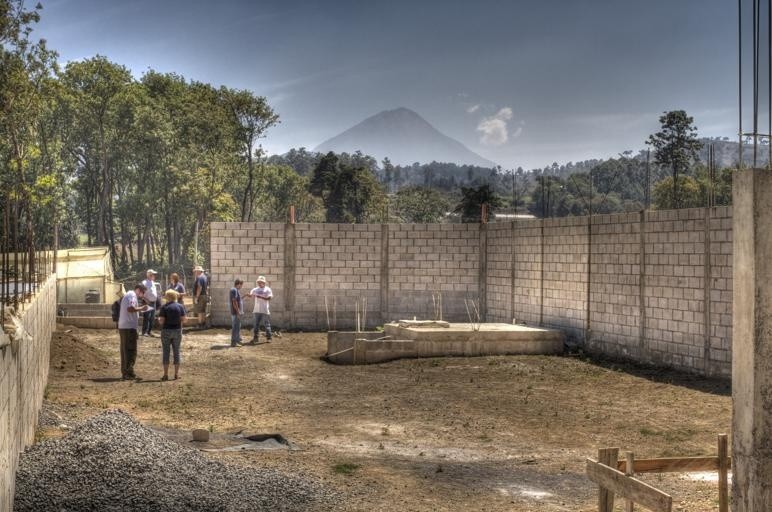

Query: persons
167 273 186 305
247 276 273 344
227 277 249 347
192 266 207 326
139 269 158 336
158 289 188 381
117 284 147 380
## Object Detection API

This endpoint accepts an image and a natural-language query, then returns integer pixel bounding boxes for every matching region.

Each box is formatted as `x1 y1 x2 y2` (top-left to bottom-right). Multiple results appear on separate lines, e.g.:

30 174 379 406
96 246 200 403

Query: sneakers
231 338 272 347
121 372 182 382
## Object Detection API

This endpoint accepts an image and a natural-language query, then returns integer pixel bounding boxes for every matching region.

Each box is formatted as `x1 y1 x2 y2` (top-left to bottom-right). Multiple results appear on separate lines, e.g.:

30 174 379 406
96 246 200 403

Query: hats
256 276 266 283
192 266 204 272
147 269 157 275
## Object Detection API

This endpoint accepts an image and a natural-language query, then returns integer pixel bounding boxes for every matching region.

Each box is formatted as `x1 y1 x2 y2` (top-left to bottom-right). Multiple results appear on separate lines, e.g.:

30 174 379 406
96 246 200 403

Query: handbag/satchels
112 297 123 322
